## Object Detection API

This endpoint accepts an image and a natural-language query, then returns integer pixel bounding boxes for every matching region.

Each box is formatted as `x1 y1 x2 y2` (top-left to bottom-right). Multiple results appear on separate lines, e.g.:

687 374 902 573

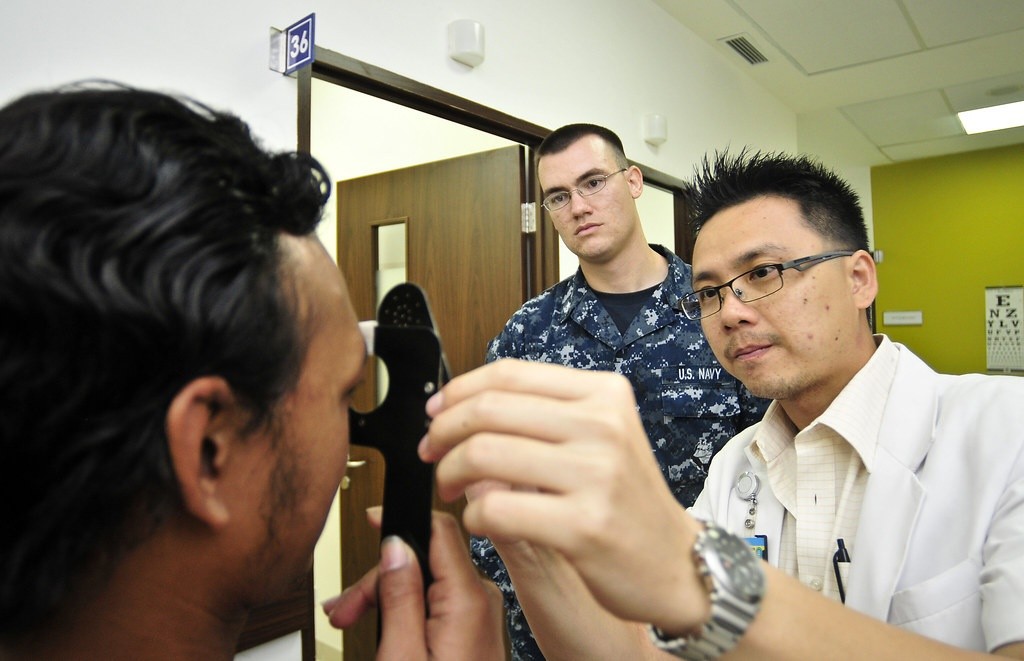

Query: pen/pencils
834 538 850 605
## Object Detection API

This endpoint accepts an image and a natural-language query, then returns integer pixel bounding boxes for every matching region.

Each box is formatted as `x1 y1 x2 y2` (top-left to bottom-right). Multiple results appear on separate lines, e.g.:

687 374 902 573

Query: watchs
645 517 767 659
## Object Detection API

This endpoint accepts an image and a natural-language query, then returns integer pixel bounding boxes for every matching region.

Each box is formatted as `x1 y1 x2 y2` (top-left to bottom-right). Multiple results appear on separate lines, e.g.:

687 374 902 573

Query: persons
417 154 1024 661
463 122 776 661
462 392 468 395
0 87 512 661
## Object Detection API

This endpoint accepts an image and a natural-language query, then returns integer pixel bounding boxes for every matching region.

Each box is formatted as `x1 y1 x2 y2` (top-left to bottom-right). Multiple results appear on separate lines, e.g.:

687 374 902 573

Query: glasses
679 250 852 320
540 168 628 212
